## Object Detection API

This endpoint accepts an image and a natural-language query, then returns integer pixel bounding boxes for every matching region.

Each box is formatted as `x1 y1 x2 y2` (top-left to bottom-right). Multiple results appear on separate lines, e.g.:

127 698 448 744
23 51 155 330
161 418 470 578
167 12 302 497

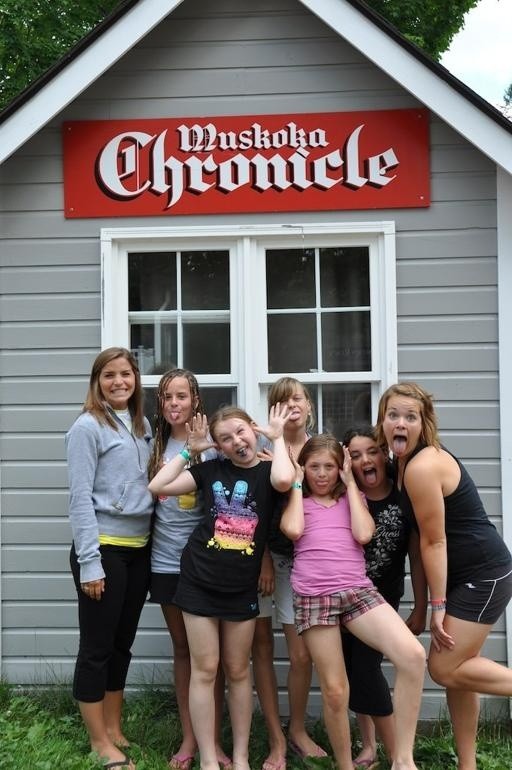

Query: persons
372 384 512 769
148 369 232 770
253 376 426 770
63 345 153 770
147 402 295 770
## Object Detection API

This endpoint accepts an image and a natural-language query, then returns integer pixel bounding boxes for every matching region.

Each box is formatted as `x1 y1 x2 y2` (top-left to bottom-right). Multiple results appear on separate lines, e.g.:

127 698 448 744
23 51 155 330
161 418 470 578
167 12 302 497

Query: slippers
288 740 328 760
353 760 380 769
104 753 134 770
262 758 287 770
219 754 231 770
171 754 195 768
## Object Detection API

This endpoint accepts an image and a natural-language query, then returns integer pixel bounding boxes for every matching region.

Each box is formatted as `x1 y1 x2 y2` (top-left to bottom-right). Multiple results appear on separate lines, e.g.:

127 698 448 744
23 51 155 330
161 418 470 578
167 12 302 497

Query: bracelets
180 450 192 462
430 599 447 610
